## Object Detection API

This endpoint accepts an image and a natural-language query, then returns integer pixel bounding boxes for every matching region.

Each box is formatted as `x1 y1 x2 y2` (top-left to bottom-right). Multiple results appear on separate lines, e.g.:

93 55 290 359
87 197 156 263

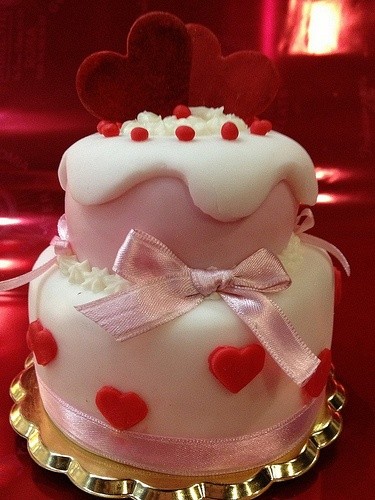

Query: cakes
20 8 337 471
